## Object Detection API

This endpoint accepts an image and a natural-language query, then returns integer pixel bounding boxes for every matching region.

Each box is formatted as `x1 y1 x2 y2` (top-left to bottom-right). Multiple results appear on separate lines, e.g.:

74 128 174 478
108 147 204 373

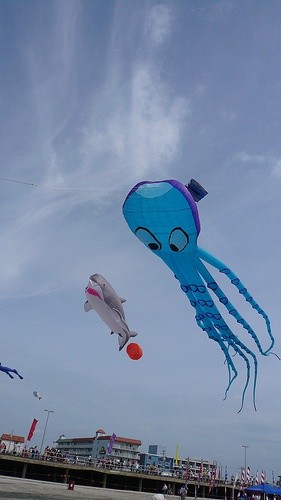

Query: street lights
37 409 55 459
242 445 248 485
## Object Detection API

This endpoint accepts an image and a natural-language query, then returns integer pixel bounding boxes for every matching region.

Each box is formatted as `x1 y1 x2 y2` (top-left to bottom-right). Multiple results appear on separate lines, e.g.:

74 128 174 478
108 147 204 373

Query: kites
33 391 42 400
121 179 281 414
0 362 24 380
83 273 143 360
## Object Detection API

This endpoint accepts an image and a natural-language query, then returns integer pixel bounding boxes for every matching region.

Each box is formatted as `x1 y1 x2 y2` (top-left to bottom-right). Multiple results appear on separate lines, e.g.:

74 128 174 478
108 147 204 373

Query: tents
244 482 281 500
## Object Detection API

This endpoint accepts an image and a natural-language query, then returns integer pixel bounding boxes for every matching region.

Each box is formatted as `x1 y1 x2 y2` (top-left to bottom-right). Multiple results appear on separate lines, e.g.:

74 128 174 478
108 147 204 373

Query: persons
162 484 172 497
237 491 278 500
224 477 253 487
0 443 158 476
180 485 187 500
